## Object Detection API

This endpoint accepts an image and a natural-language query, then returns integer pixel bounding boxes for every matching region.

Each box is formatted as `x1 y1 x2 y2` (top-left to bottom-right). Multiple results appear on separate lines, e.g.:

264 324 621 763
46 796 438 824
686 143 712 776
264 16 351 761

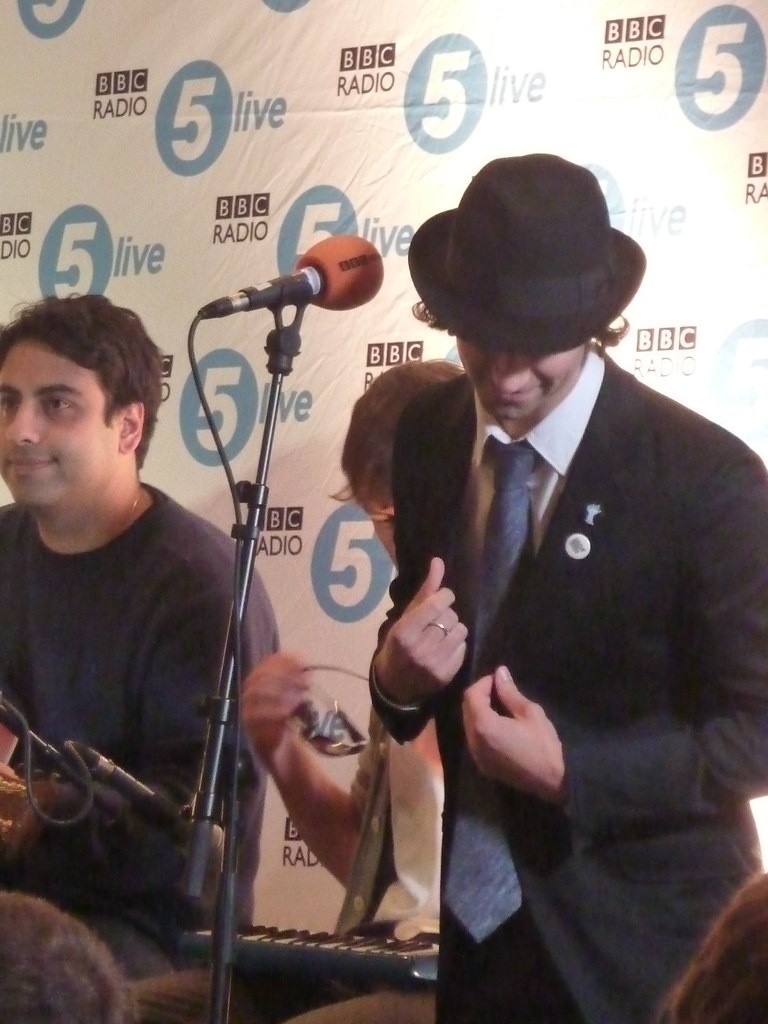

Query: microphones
199 235 383 319
74 739 179 829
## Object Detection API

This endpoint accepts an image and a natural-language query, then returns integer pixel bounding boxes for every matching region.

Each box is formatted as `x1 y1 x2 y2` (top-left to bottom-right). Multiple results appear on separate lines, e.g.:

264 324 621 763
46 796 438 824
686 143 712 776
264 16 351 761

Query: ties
443 434 543 944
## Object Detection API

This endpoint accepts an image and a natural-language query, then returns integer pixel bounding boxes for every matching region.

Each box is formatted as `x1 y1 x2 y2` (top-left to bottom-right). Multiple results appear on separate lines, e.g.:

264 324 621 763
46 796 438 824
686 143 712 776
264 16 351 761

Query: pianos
177 922 442 981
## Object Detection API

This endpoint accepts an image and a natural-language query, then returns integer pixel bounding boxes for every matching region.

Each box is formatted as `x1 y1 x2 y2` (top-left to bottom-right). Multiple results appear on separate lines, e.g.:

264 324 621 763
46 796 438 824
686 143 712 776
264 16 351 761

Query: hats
407 153 646 356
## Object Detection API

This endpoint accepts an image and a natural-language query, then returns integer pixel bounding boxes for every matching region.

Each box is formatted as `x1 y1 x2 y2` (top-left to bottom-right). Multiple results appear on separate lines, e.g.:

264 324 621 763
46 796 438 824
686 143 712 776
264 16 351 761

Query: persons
0 891 142 1024
0 292 279 986
369 153 768 1024
123 358 469 1024
650 872 768 1024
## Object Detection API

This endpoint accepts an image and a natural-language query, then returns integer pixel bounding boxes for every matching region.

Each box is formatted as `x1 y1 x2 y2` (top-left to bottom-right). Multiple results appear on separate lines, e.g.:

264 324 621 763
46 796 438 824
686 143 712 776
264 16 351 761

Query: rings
427 622 449 637
